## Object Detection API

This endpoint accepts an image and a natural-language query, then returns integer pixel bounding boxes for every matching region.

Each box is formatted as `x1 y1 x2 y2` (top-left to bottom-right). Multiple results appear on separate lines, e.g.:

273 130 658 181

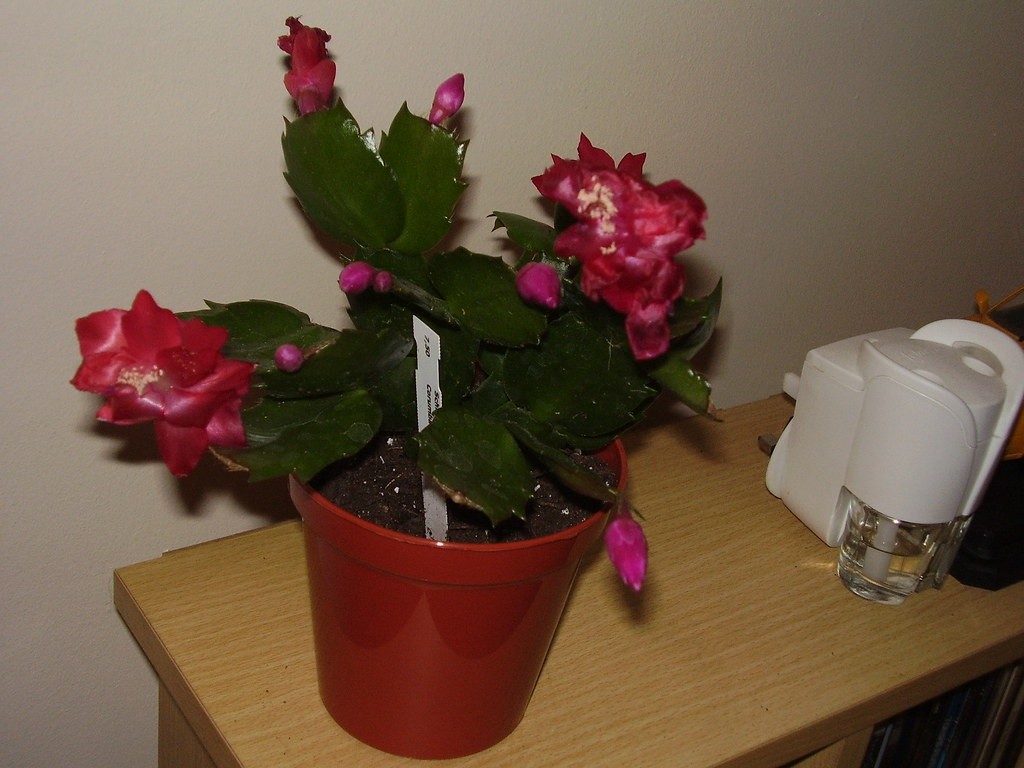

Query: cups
841 503 976 605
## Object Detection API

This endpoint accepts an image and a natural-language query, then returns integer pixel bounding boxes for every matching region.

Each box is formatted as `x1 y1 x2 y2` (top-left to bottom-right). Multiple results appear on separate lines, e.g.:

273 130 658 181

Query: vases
289 431 627 762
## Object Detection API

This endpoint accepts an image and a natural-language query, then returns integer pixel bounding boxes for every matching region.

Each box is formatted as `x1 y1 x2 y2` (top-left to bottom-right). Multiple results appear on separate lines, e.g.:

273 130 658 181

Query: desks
108 397 1024 768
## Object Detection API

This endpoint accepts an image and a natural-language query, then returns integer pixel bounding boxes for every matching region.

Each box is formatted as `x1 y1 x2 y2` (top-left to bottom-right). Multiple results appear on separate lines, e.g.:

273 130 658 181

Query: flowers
67 14 723 598
